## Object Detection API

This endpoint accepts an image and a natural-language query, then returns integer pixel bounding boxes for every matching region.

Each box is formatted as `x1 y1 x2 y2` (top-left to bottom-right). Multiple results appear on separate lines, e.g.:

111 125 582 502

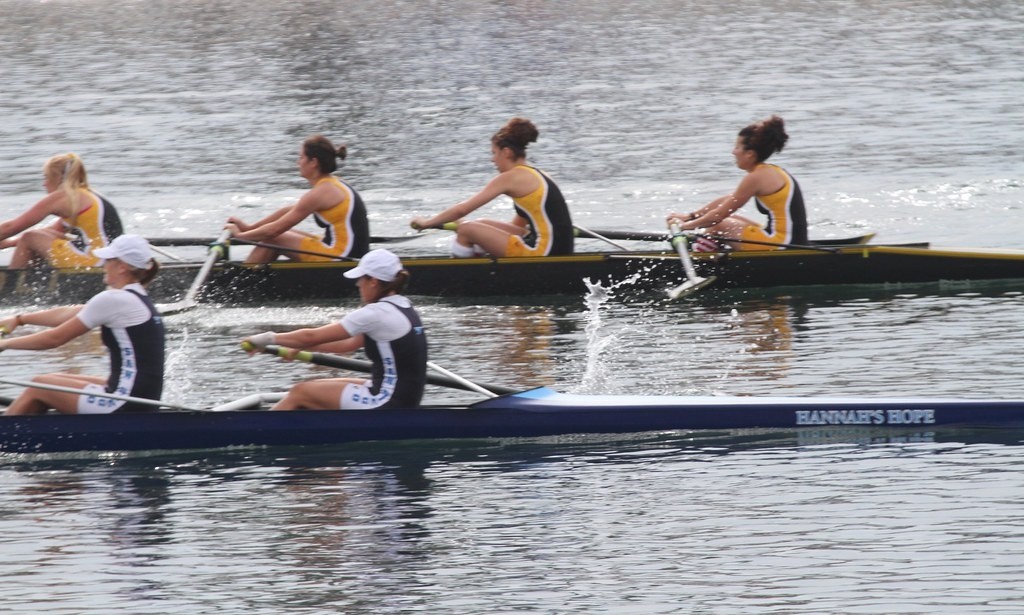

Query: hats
91 234 153 270
342 248 402 281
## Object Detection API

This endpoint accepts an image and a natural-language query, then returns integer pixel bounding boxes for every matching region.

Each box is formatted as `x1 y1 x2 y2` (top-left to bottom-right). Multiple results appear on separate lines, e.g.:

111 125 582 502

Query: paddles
668 223 718 300
410 221 877 246
147 229 428 249
240 339 525 396
154 221 235 316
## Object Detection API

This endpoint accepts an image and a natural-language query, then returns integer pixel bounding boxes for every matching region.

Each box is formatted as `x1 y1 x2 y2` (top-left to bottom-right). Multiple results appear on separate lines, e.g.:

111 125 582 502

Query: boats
1 384 1024 451
0 238 1024 307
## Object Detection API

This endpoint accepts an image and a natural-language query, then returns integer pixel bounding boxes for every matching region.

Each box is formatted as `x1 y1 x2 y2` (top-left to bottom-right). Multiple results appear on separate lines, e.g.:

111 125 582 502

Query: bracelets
16 315 23 326
691 213 695 219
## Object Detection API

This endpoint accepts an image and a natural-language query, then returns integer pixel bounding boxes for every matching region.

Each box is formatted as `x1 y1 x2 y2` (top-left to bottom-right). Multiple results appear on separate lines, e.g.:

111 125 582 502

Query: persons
223 136 370 263
239 249 428 410
1 153 123 270
0 233 165 416
410 117 575 257
666 115 808 251
